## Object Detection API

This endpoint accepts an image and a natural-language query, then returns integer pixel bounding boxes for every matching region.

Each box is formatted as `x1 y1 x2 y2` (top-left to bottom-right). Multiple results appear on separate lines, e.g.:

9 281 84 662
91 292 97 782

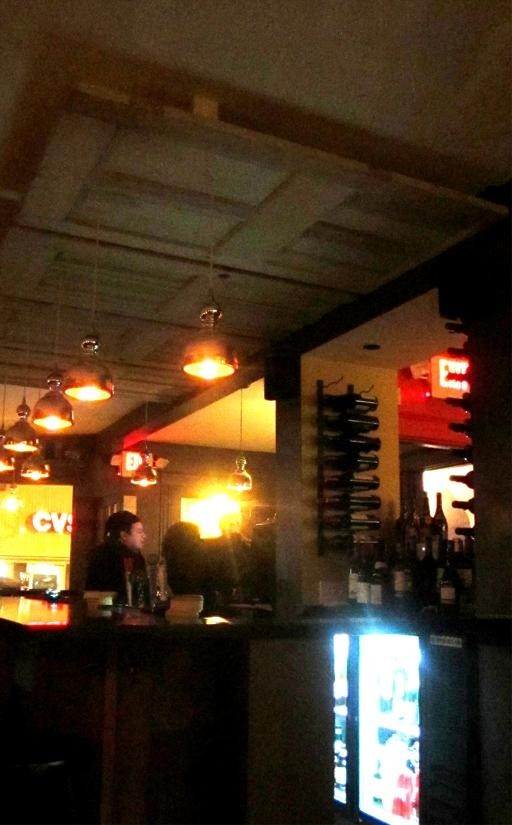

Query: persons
162 519 221 612
86 510 151 606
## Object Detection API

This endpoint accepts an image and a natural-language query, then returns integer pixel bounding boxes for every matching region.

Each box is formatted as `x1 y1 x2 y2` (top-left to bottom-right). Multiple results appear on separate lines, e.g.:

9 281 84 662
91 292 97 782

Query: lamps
224 374 251 490
130 402 159 488
31 279 75 431
4 307 41 453
180 189 240 380
20 452 50 479
2 377 15 473
61 211 114 403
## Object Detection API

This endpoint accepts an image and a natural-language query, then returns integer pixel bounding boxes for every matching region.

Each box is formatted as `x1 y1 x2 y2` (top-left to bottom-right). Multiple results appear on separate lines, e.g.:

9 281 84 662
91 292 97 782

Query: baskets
84 591 114 607
85 607 112 618
165 610 199 622
169 594 204 612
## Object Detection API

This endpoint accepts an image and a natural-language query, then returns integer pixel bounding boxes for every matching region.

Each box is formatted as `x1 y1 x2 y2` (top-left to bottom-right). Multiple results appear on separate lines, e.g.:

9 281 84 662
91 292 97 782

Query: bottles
320 454 379 470
397 489 447 560
322 512 380 531
321 492 381 511
321 535 384 554
347 535 480 614
321 472 380 489
322 392 378 411
321 413 379 432
322 434 381 451
